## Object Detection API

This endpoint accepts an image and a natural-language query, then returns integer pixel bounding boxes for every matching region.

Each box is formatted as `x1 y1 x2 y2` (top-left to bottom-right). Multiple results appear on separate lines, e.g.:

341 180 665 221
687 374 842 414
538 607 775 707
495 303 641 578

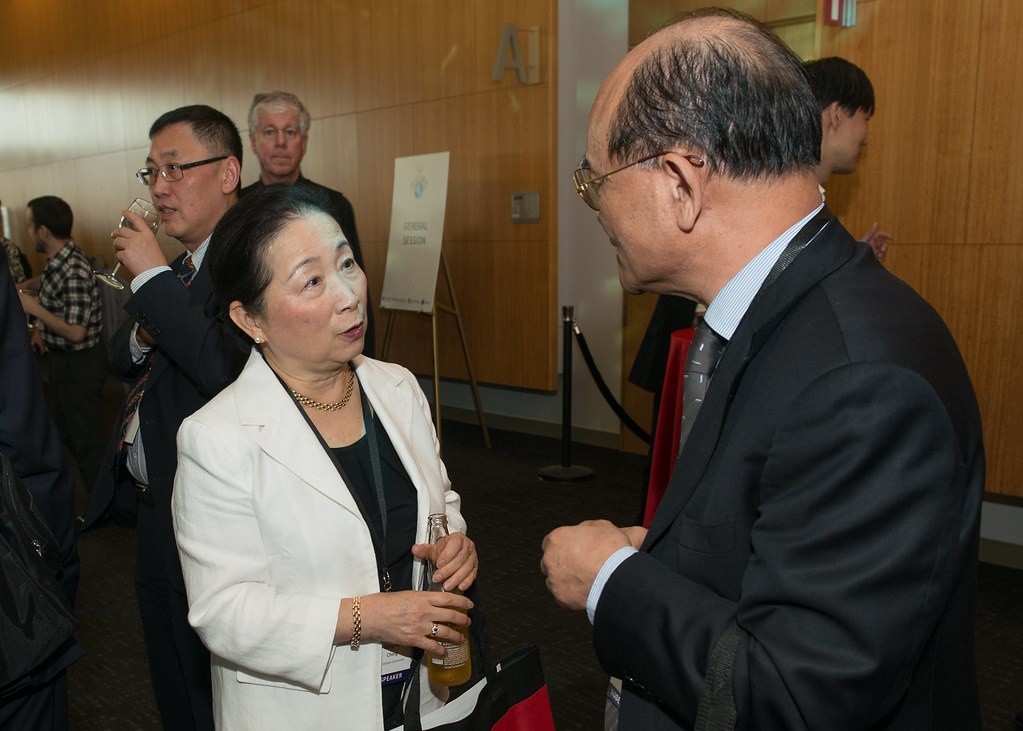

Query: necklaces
289 359 355 411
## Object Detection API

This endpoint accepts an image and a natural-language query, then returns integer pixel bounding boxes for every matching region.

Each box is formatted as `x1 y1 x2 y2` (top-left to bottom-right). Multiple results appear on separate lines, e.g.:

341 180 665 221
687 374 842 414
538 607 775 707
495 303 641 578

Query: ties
114 253 196 466
674 319 724 460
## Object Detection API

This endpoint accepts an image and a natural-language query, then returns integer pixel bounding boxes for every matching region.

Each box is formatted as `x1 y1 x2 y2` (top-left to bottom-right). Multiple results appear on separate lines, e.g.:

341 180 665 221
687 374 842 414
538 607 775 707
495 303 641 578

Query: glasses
135 153 229 187
571 149 705 213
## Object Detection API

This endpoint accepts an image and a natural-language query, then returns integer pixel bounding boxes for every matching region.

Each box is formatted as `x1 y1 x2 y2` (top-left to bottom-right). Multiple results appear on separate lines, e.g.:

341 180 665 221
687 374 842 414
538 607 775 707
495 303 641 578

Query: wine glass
19 289 41 330
93 199 164 289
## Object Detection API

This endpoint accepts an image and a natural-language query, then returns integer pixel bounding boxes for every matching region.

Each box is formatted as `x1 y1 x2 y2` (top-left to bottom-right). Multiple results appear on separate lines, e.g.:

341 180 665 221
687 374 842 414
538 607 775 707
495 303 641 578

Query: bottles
422 512 472 685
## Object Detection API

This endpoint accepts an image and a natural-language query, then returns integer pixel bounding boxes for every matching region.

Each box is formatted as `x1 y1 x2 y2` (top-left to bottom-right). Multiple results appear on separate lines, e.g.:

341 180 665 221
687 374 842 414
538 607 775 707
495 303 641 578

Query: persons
633 55 891 447
98 101 269 726
171 182 485 728
535 8 997 731
0 236 84 731
236 91 375 362
0 234 43 352
12 194 108 529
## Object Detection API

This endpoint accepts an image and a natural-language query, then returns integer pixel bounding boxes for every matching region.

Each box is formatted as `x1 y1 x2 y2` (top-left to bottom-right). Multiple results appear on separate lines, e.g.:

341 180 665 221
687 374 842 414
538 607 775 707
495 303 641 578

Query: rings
429 622 440 637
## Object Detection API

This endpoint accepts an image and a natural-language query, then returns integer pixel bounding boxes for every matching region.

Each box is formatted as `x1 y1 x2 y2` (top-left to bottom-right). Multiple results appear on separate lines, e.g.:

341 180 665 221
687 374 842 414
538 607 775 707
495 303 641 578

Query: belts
136 485 152 507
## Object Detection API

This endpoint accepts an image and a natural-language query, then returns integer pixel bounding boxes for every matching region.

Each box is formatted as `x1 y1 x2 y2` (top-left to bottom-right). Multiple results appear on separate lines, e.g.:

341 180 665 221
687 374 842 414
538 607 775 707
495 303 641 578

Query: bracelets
349 592 363 649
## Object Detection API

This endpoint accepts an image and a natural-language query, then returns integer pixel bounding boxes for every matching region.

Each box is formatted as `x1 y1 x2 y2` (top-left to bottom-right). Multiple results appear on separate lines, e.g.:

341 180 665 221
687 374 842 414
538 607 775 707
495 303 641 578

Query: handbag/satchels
382 642 557 731
